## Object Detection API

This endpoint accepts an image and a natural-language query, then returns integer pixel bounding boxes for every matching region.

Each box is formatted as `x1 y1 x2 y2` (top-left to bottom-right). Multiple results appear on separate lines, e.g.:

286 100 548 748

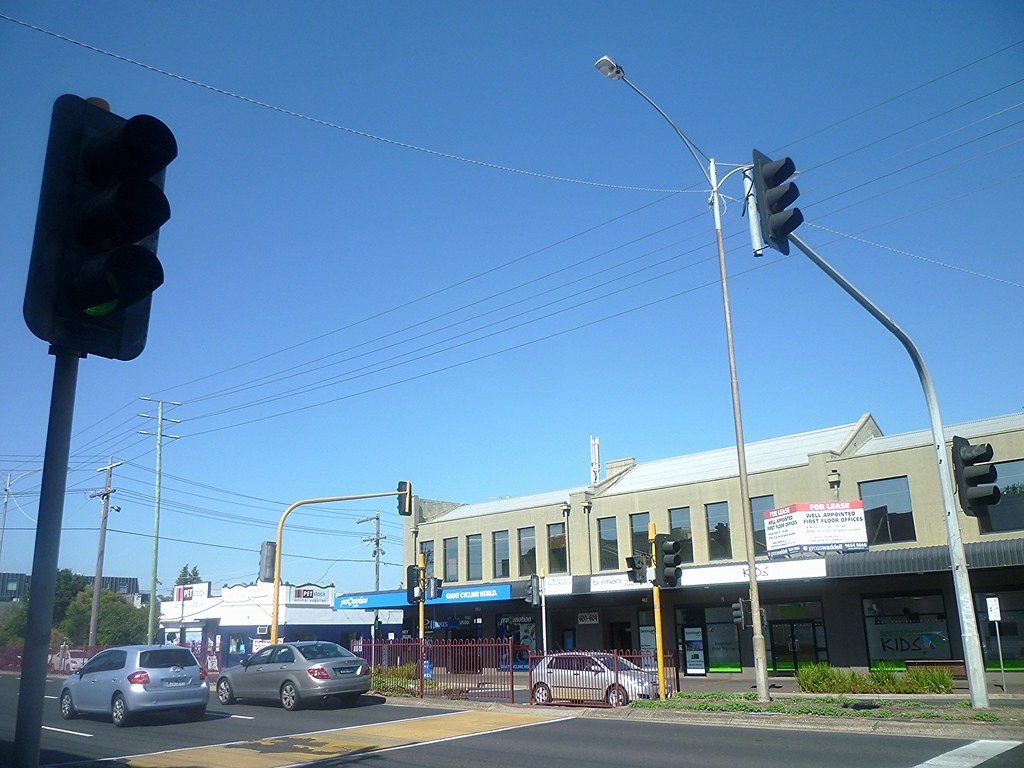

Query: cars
529 650 673 710
59 644 211 728
216 640 372 711
48 649 91 674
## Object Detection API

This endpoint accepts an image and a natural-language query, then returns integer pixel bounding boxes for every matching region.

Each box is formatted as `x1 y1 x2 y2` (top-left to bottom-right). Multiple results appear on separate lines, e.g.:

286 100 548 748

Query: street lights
593 54 773 704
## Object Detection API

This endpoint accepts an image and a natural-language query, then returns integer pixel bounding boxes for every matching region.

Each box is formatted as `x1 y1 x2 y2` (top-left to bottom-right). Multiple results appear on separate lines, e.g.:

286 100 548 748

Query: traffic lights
731 597 749 631
624 555 647 583
524 574 540 608
427 577 443 600
752 147 805 256
953 435 1001 517
22 93 179 362
654 533 683 588
